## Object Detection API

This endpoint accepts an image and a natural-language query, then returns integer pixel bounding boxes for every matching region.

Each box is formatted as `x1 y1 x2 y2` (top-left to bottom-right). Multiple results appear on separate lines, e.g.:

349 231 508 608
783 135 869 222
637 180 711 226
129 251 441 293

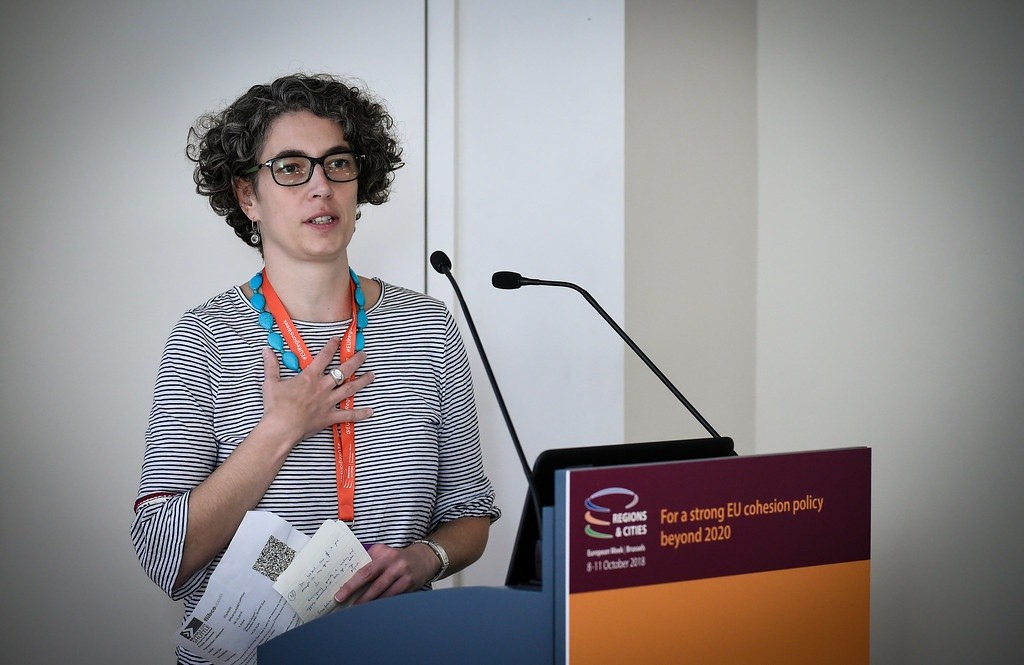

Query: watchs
411 536 450 583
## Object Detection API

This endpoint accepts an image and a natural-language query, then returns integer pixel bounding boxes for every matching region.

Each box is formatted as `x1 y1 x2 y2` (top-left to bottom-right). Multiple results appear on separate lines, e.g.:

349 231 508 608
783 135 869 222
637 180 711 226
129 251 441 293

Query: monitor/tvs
503 436 732 587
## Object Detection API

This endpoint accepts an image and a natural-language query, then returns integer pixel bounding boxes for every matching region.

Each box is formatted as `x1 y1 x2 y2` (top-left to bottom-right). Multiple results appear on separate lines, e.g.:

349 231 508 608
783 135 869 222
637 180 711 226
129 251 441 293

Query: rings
330 369 344 386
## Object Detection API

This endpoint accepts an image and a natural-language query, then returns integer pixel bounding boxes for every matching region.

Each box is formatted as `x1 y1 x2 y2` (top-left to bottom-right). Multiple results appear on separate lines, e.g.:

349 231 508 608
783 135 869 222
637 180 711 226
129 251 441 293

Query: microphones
429 250 544 541
490 270 738 457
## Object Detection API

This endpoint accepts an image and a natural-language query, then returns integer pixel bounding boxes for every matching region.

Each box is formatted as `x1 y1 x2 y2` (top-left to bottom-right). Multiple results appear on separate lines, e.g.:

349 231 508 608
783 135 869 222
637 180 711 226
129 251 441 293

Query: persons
130 73 502 665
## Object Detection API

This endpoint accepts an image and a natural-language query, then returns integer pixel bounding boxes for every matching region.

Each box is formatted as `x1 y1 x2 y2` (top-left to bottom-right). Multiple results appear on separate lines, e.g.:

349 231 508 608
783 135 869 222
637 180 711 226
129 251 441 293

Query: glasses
241 151 366 186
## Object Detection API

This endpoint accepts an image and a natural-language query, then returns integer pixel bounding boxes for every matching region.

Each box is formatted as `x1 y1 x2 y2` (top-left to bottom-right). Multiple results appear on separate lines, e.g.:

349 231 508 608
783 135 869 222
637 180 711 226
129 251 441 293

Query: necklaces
249 264 368 374
